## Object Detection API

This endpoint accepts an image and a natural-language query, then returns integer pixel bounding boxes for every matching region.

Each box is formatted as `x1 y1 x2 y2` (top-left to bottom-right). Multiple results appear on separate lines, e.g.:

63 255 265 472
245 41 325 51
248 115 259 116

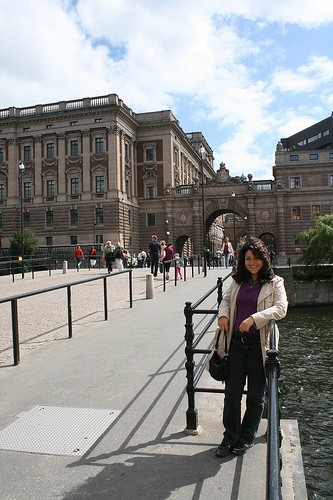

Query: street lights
232 190 236 253
19 161 25 280
198 144 207 277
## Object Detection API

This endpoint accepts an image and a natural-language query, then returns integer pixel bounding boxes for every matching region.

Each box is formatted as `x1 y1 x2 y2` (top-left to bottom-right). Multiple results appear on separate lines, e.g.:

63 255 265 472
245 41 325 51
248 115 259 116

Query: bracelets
148 252 149 253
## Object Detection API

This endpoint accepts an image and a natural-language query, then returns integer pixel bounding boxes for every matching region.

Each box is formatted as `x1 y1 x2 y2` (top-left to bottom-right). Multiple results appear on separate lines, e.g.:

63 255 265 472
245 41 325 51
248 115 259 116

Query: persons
148 235 162 279
105 241 115 274
174 253 184 280
140 249 147 267
74 246 85 268
206 236 288 459
163 242 174 280
122 250 127 268
113 242 124 272
137 250 142 264
89 246 97 269
159 240 167 273
270 251 275 263
220 237 233 268
146 253 151 267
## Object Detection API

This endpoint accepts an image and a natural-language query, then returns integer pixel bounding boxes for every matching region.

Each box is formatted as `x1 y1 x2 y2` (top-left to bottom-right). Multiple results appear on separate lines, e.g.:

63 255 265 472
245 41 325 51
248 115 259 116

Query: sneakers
215 438 254 457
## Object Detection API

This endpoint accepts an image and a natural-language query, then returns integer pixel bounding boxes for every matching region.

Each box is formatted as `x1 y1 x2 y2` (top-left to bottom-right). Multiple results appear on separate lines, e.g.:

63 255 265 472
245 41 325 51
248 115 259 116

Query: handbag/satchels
208 351 228 382
